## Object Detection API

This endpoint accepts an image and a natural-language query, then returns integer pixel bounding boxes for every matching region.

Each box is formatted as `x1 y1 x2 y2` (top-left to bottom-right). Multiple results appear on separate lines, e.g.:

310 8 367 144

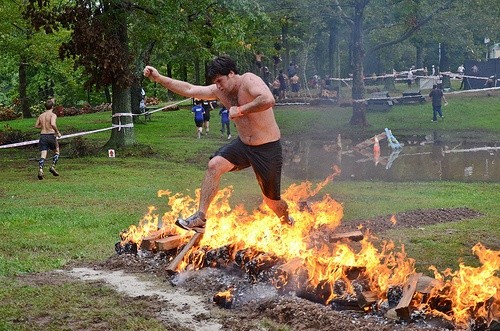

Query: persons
35 100 61 180
252 51 301 100
429 85 448 123
191 98 214 138
483 76 496 87
143 55 296 234
218 105 231 141
456 63 466 76
408 68 414 88
274 37 287 61
308 73 326 90
432 64 440 76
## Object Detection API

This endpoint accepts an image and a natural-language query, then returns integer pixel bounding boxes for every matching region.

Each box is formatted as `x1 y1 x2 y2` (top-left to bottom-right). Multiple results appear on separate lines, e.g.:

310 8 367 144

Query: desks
367 92 398 111
403 90 426 105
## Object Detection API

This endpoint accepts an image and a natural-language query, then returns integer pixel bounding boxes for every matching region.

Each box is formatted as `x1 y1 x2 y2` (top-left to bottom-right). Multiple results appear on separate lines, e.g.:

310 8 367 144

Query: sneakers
289 216 294 228
175 212 207 234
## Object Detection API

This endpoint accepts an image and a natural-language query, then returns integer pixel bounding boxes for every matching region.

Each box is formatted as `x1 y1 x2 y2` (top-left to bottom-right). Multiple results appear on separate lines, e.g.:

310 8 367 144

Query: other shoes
206 132 209 135
431 120 437 122
49 167 59 176
442 116 444 118
221 134 223 138
200 132 203 135
228 135 231 139
38 171 44 180
